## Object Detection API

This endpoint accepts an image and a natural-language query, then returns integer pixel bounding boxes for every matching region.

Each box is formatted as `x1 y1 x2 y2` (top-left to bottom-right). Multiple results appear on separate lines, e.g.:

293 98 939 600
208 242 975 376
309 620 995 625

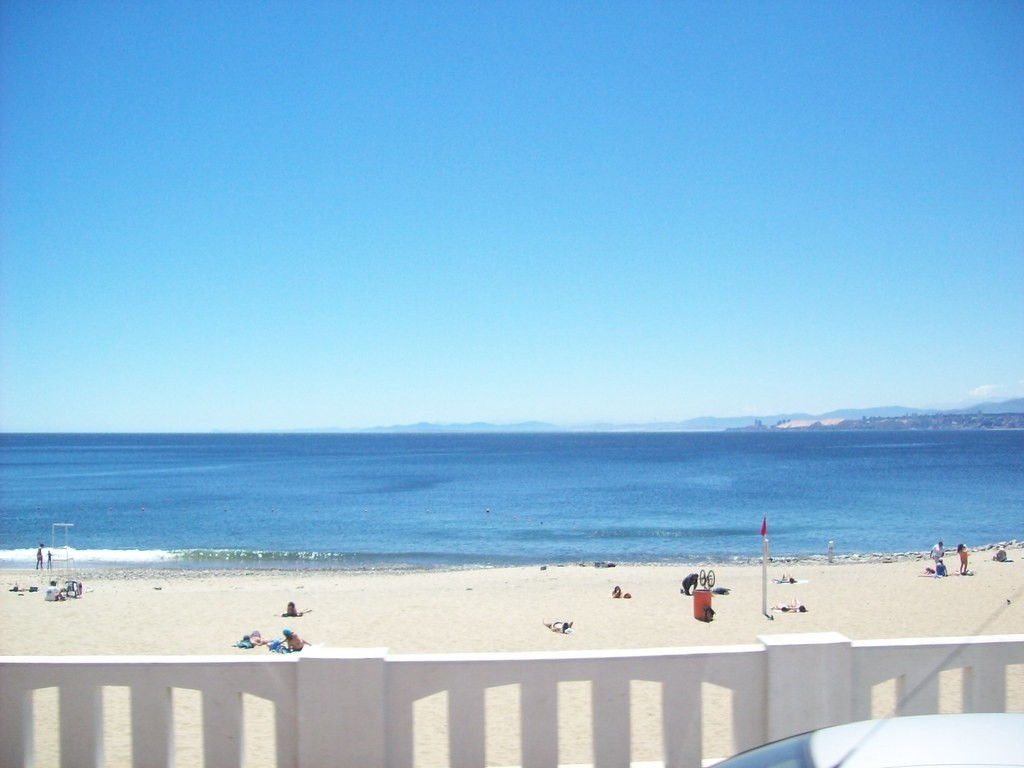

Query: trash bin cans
693 590 712 620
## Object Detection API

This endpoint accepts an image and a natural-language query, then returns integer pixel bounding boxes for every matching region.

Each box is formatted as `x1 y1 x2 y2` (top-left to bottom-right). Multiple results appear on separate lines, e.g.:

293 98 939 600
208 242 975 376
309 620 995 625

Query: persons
680 574 699 596
611 587 623 599
45 581 66 601
774 573 806 613
925 542 968 576
36 544 53 570
544 619 573 633
993 545 1007 561
242 601 312 652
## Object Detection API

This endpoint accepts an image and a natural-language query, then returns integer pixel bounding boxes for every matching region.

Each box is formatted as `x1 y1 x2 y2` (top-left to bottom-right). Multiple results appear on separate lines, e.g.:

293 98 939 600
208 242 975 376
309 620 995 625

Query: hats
939 558 943 561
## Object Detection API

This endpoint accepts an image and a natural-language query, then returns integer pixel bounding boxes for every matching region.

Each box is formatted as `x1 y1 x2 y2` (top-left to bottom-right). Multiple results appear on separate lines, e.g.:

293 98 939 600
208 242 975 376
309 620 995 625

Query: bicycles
699 569 716 598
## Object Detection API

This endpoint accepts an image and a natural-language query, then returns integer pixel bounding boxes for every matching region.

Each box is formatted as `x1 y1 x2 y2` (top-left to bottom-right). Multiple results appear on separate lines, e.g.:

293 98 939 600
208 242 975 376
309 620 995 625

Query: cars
703 710 1024 768
